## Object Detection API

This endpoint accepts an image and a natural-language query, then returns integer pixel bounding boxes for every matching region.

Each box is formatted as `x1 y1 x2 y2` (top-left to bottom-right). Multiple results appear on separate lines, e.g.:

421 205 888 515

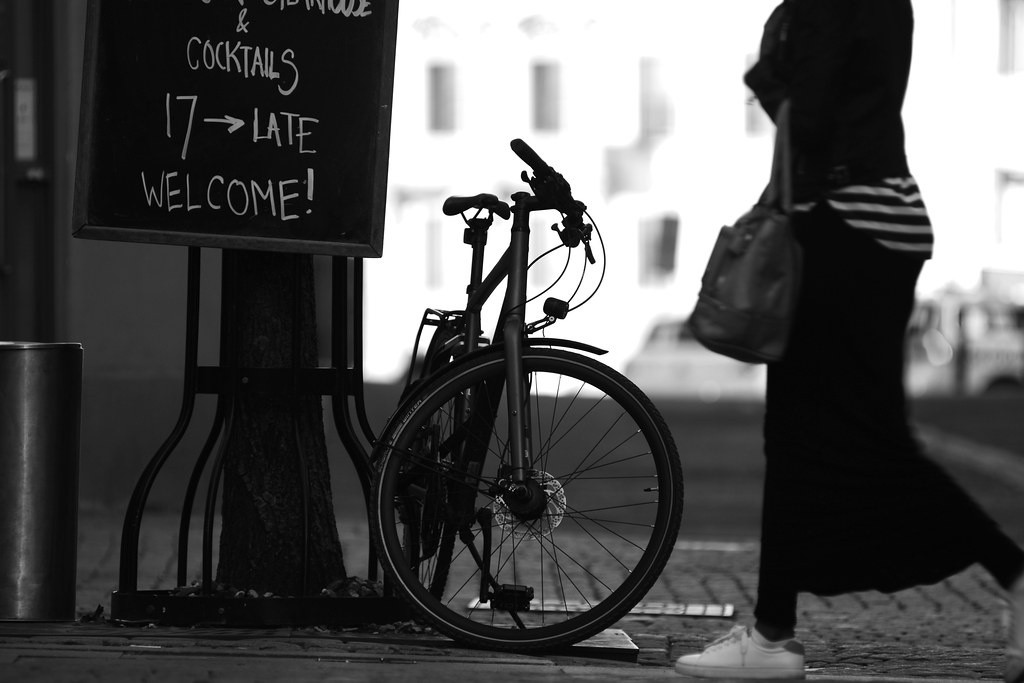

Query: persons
676 0 1024 681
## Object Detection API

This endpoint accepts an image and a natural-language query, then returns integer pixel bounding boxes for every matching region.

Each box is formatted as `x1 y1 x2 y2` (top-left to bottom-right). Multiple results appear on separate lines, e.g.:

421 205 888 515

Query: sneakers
675 624 805 680
999 572 1024 681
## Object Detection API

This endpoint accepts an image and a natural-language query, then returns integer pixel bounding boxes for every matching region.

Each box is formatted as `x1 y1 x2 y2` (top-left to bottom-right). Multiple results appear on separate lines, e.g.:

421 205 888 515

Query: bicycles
365 138 685 655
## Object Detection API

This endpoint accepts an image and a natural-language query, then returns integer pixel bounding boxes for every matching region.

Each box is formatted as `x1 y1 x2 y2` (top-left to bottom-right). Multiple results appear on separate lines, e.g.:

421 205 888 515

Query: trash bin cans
0 345 85 631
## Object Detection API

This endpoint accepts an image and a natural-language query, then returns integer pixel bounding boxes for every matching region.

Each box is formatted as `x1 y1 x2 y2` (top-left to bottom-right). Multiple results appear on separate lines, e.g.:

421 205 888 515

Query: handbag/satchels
688 206 794 362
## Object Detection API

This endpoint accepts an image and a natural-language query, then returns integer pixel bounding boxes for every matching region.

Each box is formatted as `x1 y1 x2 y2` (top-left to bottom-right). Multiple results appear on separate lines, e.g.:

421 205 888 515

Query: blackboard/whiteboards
74 0 400 257
